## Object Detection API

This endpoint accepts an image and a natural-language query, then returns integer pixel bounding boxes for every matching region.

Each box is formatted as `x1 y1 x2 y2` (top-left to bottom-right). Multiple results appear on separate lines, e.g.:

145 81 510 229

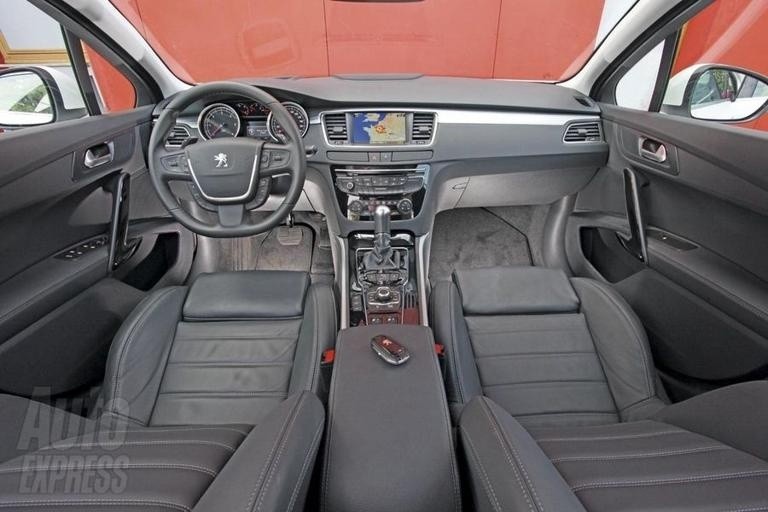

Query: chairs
429 265 768 512
0 268 337 512
